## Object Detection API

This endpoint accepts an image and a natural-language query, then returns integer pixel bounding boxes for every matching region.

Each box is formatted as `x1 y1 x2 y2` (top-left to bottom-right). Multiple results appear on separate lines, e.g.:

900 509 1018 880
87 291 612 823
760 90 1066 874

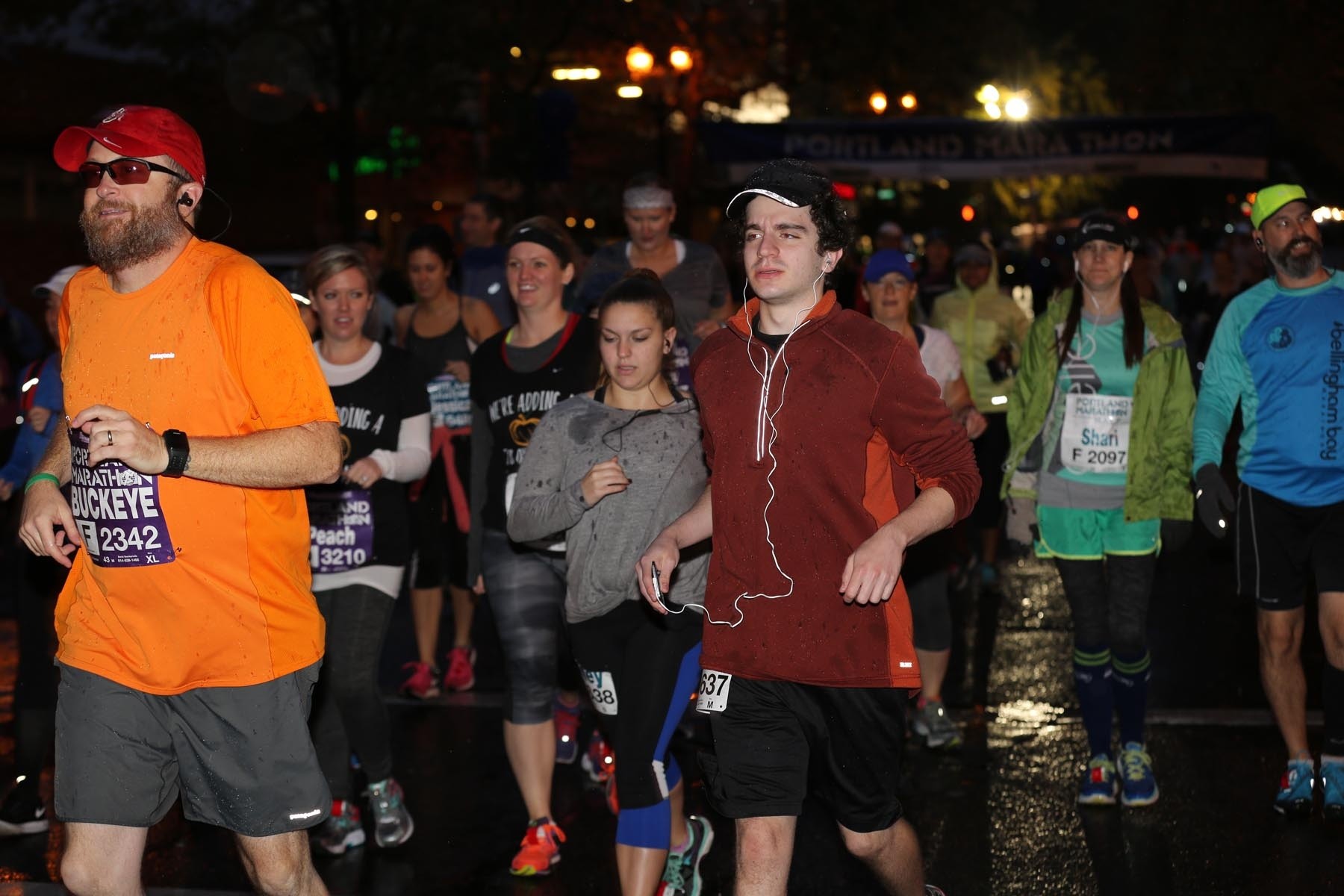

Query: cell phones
650 562 666 605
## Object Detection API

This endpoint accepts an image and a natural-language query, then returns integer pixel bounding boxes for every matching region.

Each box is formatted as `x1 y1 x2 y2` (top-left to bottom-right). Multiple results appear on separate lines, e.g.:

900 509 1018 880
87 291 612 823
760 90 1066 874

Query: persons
635 159 981 896
278 170 736 896
858 183 1344 809
0 264 94 835
17 103 344 895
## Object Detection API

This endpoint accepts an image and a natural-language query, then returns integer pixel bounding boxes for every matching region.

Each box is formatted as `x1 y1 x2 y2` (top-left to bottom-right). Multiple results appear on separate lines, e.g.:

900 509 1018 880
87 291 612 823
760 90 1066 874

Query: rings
361 476 368 480
108 431 112 445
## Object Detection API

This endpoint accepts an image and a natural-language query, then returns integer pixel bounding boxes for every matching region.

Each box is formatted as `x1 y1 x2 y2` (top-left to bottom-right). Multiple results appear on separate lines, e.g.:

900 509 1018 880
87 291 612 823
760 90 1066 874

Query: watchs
162 429 189 479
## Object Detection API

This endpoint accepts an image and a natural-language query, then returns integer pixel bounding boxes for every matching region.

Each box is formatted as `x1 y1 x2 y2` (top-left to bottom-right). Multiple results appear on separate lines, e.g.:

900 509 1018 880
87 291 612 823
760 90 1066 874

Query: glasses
78 156 191 188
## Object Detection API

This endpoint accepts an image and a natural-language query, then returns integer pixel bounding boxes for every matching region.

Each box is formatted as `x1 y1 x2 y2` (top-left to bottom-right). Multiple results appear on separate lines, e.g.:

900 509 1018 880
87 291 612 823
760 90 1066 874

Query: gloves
1194 464 1236 536
1006 496 1038 546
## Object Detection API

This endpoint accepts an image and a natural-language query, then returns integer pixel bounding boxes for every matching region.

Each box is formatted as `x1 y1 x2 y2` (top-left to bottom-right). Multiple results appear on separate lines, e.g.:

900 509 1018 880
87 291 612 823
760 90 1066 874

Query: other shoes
0 792 49 835
444 645 477 692
581 729 615 782
555 694 582 763
401 661 441 700
913 699 965 747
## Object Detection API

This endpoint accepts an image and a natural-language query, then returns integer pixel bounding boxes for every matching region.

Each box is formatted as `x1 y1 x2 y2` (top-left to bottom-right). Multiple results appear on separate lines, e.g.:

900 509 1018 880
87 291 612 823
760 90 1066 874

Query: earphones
1123 261 1129 273
827 260 832 265
180 193 193 206
1074 260 1081 271
1256 239 1264 249
665 339 670 347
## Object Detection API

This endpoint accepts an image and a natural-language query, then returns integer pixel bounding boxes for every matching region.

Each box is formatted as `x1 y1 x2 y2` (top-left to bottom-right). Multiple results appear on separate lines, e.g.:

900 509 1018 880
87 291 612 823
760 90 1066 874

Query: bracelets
24 473 60 495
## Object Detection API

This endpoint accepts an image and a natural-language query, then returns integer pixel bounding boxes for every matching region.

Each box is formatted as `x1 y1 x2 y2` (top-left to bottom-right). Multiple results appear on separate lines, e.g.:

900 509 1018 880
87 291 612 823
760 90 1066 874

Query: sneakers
510 817 566 876
367 775 414 846
657 815 714 896
1118 742 1159 806
1319 754 1344 811
1078 753 1118 803
317 800 366 854
1274 749 1316 815
607 773 619 817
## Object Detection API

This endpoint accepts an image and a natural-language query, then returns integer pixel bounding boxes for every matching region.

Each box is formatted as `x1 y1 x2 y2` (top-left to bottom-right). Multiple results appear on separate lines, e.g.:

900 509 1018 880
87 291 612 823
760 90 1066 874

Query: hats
863 248 915 283
1250 183 1305 230
1072 215 1129 249
726 158 833 219
53 106 206 189
